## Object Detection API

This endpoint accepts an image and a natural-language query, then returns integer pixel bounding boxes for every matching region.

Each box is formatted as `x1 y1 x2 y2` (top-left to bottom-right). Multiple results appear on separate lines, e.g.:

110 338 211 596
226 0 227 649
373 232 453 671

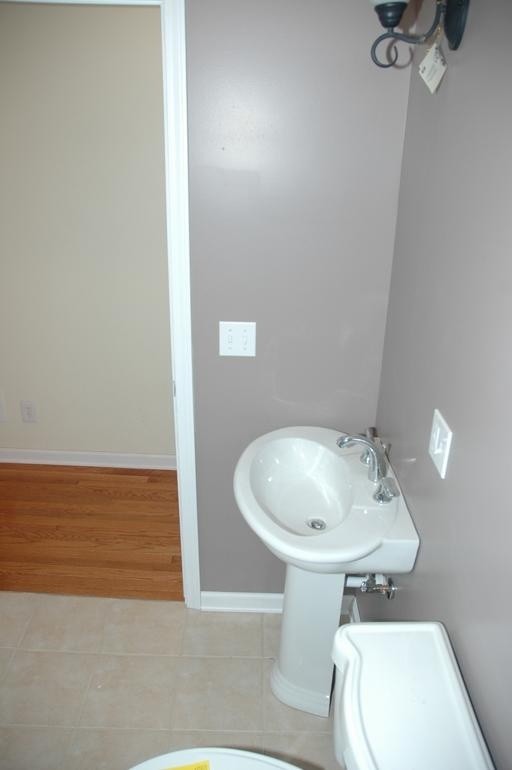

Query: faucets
336 433 387 482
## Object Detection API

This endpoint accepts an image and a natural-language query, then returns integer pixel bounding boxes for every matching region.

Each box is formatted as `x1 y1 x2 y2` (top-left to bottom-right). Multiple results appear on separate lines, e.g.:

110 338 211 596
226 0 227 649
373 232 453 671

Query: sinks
233 426 421 576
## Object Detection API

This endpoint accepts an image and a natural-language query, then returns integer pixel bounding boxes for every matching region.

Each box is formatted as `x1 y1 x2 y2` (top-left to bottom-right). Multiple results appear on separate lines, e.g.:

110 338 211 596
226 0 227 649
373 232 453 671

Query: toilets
128 621 498 768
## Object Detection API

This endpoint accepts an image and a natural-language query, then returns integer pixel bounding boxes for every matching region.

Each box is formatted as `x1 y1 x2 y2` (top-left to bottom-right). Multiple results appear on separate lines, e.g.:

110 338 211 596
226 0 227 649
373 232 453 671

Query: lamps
369 0 471 68
